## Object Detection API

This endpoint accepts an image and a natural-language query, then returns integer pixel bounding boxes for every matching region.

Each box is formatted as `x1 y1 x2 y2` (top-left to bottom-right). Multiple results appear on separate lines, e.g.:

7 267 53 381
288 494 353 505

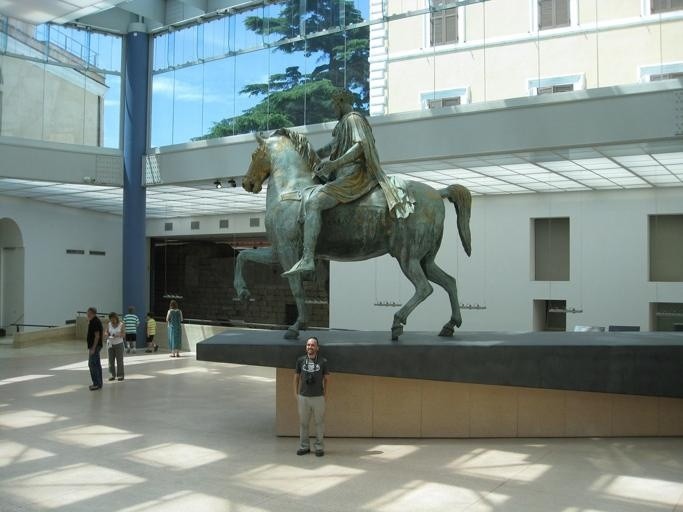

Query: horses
232 129 472 340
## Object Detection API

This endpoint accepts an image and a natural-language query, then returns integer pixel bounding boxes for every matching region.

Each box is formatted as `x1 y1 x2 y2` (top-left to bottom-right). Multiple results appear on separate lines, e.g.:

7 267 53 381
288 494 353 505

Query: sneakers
144 349 152 353
154 344 158 352
316 449 324 456
89 385 102 390
296 449 310 455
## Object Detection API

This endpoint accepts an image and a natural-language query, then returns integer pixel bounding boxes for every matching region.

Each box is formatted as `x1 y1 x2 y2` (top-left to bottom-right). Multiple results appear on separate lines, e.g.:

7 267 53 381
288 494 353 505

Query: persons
87 307 103 391
279 86 417 279
144 311 159 353
292 337 331 457
166 300 184 357
104 311 126 380
122 306 141 353
